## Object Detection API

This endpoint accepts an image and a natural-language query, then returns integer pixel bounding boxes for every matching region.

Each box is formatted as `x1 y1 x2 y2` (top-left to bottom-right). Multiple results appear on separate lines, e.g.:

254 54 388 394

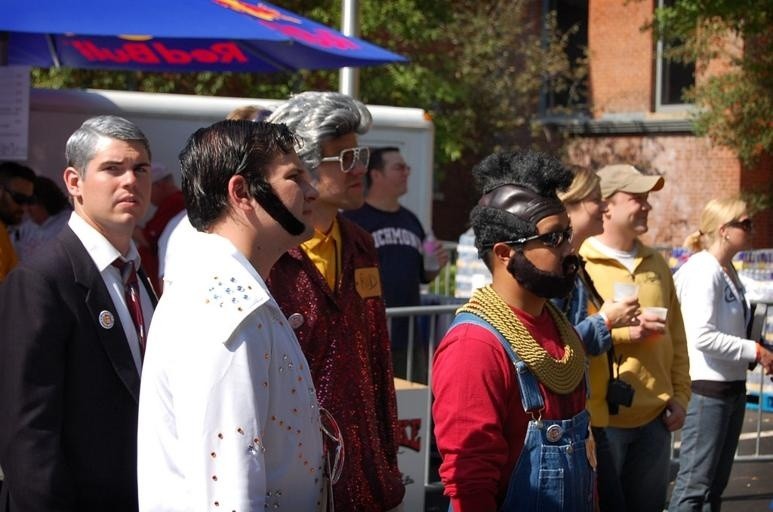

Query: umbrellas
0 0 410 71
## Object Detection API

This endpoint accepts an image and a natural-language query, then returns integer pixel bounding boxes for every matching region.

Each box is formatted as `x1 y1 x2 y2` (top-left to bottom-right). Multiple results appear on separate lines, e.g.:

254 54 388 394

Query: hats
596 164 666 198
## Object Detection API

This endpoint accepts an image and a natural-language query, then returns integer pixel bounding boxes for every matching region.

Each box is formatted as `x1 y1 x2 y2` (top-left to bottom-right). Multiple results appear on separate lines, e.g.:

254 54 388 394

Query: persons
1 93 773 512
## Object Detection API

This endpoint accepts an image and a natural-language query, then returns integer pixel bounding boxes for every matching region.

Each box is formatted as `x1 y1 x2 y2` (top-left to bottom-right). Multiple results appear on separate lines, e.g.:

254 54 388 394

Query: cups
641 306 667 325
615 283 638 305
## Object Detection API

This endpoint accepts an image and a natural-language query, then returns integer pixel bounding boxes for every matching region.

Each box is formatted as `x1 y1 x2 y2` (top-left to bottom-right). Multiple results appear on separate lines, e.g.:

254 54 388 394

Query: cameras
606 379 634 415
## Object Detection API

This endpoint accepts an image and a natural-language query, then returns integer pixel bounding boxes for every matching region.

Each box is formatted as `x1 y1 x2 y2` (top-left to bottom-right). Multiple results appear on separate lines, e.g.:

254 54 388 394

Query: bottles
737 247 773 281
421 228 438 272
655 247 688 273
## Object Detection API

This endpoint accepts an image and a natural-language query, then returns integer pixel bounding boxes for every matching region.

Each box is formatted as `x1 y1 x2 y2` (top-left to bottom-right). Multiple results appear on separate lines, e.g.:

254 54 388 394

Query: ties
113 258 148 360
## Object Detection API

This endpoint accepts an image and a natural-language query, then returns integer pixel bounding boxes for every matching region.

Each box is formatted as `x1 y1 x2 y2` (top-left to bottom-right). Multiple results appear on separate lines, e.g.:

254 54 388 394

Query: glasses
729 218 752 232
321 146 373 172
503 226 572 249
315 406 346 484
